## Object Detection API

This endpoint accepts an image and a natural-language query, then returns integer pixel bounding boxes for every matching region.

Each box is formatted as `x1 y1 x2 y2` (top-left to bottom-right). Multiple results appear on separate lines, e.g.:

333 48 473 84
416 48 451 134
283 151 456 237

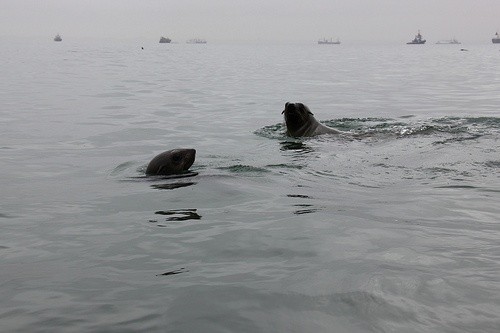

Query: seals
146 149 196 174
281 102 342 137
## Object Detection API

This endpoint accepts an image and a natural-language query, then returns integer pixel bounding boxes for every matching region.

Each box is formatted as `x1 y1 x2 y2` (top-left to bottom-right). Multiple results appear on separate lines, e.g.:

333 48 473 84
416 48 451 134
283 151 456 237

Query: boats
492 33 500 44
406 29 426 45
318 38 341 45
54 33 62 42
159 36 171 44
